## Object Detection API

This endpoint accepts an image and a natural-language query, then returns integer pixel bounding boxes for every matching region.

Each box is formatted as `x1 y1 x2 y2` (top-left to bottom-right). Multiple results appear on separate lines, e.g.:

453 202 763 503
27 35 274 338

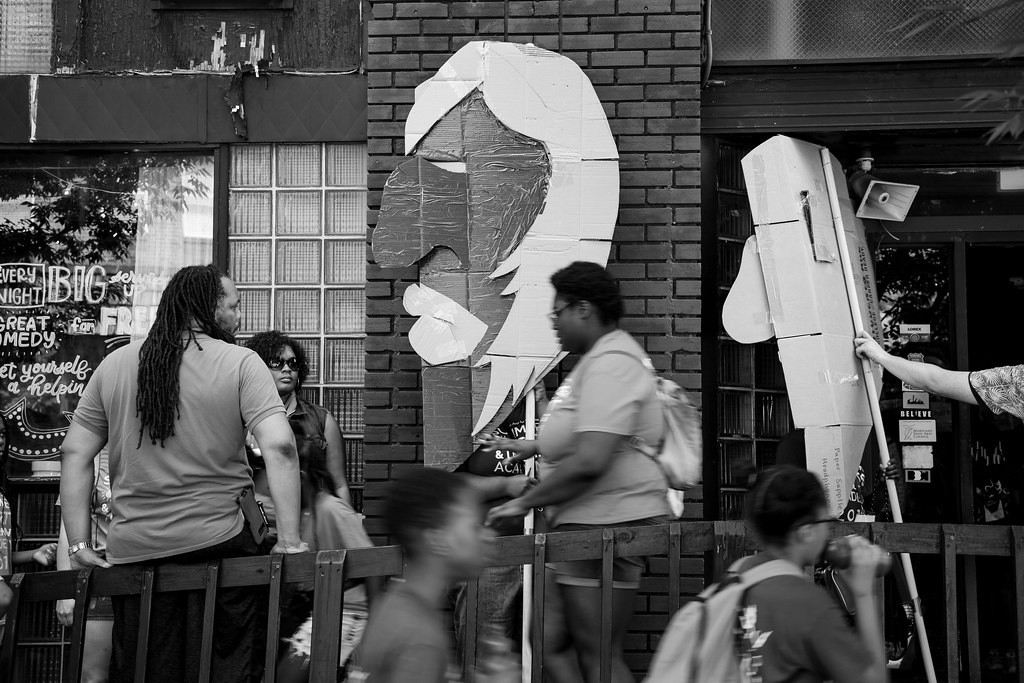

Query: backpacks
590 351 702 488
640 553 812 683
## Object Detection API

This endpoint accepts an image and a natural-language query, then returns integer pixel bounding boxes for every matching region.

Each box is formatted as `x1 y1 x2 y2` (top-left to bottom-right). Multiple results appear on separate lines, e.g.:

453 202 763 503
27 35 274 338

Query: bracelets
68 542 91 557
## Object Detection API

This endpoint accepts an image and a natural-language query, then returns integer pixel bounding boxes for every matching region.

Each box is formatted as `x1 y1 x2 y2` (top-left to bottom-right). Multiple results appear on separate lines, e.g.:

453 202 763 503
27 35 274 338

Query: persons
276 438 380 683
240 330 354 683
724 330 1024 683
351 467 496 683
1 265 308 683
458 261 677 683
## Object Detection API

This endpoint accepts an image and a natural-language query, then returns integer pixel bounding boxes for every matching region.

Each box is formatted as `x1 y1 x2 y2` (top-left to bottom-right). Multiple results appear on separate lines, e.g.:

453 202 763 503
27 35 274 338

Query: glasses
269 356 303 372
548 300 584 321
797 517 843 530
